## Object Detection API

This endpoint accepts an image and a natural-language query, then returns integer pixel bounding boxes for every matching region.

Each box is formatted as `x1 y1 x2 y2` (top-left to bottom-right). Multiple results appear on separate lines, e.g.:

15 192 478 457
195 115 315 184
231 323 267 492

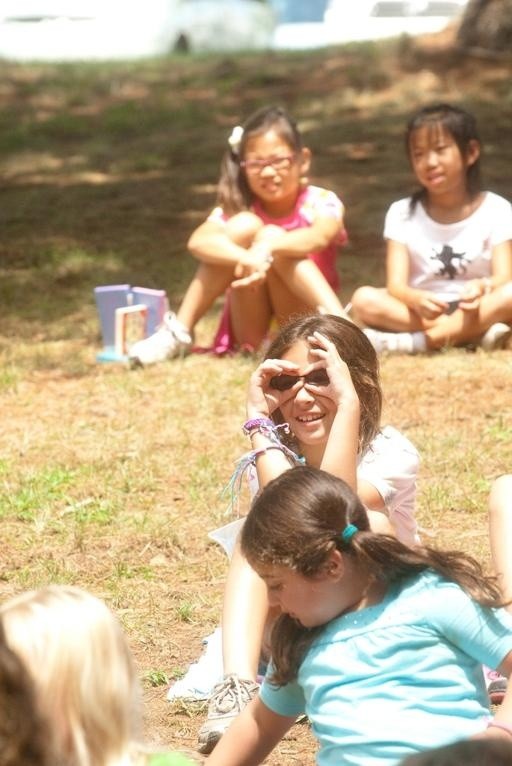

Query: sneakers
129 312 193 370
482 322 510 350
362 327 416 354
168 630 255 750
484 667 507 704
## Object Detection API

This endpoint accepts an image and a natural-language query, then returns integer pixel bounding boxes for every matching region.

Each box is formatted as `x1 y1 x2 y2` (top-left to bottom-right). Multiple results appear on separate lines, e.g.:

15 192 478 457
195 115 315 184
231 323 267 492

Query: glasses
241 157 294 173
270 369 330 391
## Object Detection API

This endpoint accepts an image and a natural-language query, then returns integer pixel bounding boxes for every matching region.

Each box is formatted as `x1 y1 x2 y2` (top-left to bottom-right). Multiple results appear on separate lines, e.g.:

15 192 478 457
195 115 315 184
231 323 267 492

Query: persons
0 584 200 766
198 463 512 766
91 283 167 367
161 315 431 753
126 103 358 361
474 468 512 703
353 97 512 357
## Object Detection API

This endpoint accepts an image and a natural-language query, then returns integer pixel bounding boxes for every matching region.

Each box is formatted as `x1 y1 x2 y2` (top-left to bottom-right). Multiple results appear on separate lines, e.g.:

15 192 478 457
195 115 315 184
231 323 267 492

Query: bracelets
237 418 307 463
483 719 511 735
221 446 305 517
